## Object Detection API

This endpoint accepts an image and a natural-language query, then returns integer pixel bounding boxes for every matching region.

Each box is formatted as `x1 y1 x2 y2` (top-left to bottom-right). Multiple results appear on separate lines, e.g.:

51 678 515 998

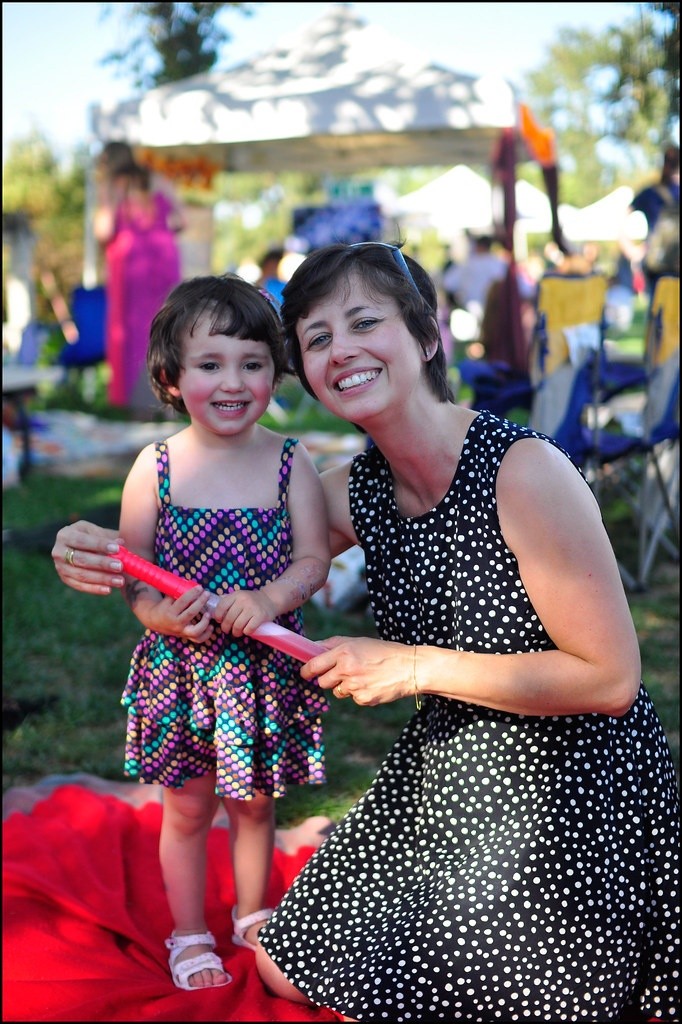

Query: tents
79 24 650 293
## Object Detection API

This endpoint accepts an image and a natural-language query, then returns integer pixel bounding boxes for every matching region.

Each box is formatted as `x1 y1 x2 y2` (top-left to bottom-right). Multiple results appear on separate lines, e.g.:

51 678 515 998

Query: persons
50 239 681 1022
445 225 535 326
118 272 332 990
2 141 680 428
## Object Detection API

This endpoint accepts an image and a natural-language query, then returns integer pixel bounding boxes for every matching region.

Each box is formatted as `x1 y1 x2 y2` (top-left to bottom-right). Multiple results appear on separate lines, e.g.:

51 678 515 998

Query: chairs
450 272 682 593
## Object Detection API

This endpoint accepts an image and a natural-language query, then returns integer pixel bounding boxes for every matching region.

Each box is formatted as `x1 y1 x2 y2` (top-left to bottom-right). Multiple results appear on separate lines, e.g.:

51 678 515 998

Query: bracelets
412 644 421 710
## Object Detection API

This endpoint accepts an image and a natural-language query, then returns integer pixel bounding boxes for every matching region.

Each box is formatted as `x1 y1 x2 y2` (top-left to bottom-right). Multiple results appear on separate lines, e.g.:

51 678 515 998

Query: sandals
231 904 276 950
164 926 232 990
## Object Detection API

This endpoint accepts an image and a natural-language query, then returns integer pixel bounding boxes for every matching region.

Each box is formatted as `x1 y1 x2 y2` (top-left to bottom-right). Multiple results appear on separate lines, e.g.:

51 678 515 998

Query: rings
65 547 75 566
337 684 346 698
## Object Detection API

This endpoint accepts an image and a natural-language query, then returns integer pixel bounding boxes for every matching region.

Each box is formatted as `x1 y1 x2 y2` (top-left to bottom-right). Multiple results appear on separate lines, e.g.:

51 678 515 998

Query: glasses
351 242 421 299
